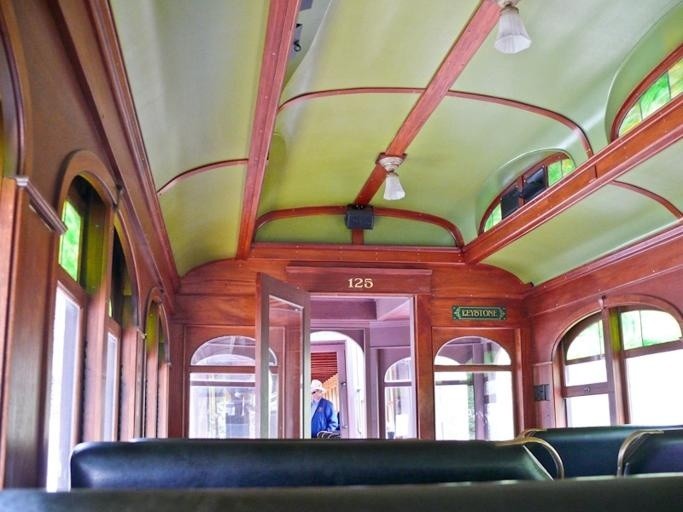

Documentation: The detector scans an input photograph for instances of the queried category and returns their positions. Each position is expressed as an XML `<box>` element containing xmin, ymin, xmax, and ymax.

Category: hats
<box><xmin>311</xmin><ymin>378</ymin><xmax>327</xmax><ymax>395</ymax></box>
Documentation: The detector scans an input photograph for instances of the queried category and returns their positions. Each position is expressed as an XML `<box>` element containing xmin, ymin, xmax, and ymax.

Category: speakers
<box><xmin>524</xmin><ymin>165</ymin><xmax>545</xmax><ymax>201</ymax></box>
<box><xmin>534</xmin><ymin>384</ymin><xmax>549</xmax><ymax>401</ymax></box>
<box><xmin>501</xmin><ymin>189</ymin><xmax>520</xmax><ymax>219</ymax></box>
<box><xmin>345</xmin><ymin>204</ymin><xmax>373</xmax><ymax>229</ymax></box>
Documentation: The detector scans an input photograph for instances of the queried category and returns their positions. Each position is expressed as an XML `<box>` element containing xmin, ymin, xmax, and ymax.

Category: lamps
<box><xmin>491</xmin><ymin>0</ymin><xmax>532</xmax><ymax>56</ymax></box>
<box><xmin>375</xmin><ymin>151</ymin><xmax>407</xmax><ymax>201</ymax></box>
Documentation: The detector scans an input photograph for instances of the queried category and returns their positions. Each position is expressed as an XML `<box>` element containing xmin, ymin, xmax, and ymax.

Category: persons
<box><xmin>308</xmin><ymin>378</ymin><xmax>339</xmax><ymax>440</ymax></box>
